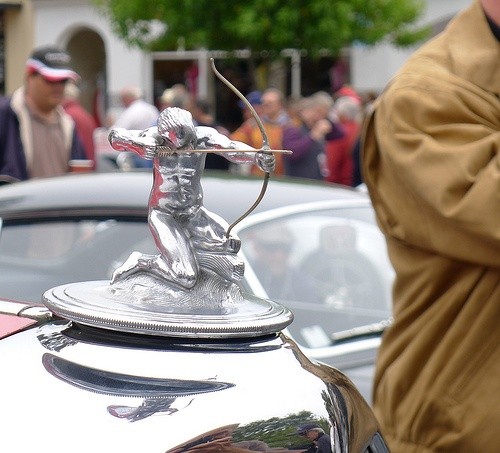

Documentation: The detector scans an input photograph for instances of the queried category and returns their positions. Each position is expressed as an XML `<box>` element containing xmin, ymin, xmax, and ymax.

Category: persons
<box><xmin>0</xmin><ymin>44</ymin><xmax>84</xmax><ymax>191</ymax></box>
<box><xmin>358</xmin><ymin>0</ymin><xmax>500</xmax><ymax>453</ymax></box>
<box><xmin>105</xmin><ymin>104</ymin><xmax>275</xmax><ymax>293</ymax></box>
<box><xmin>157</xmin><ymin>68</ymin><xmax>373</xmax><ymax>189</ymax></box>
<box><xmin>59</xmin><ymin>81</ymin><xmax>99</xmax><ymax>172</ymax></box>
<box><xmin>289</xmin><ymin>425</ymin><xmax>331</xmax><ymax>453</ymax></box>
<box><xmin>104</xmin><ymin>84</ymin><xmax>162</xmax><ymax>171</ymax></box>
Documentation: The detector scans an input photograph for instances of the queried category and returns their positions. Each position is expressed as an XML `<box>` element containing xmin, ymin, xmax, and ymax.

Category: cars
<box><xmin>0</xmin><ymin>167</ymin><xmax>398</xmax><ymax>453</ymax></box>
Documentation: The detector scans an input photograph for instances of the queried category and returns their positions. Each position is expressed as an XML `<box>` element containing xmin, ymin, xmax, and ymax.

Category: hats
<box><xmin>24</xmin><ymin>44</ymin><xmax>83</xmax><ymax>82</ymax></box>
<box><xmin>287</xmin><ymin>423</ymin><xmax>320</xmax><ymax>437</ymax></box>
<box><xmin>237</xmin><ymin>90</ymin><xmax>264</xmax><ymax>109</ymax></box>
